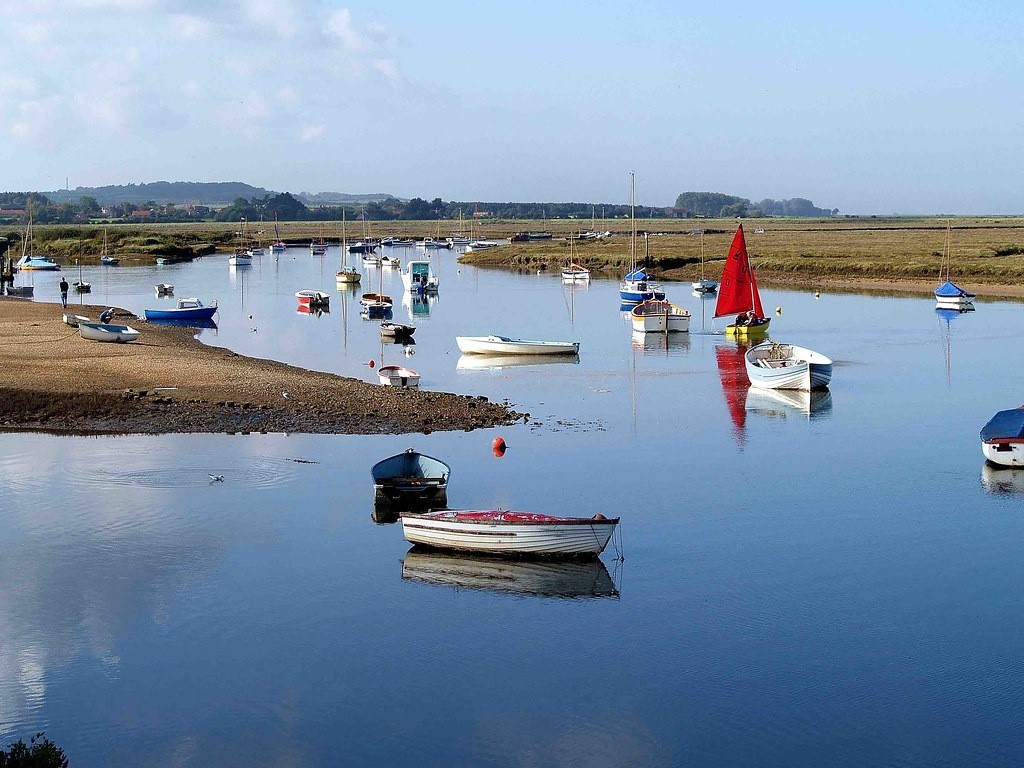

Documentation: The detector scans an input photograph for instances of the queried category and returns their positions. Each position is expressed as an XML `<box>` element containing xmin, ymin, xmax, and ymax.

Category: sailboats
<box><xmin>934</xmin><ymin>302</ymin><xmax>976</xmax><ymax>385</ymax></box>
<box><xmin>692</xmin><ymin>232</ymin><xmax>716</xmax><ymax>292</ymax></box>
<box><xmin>712</xmin><ymin>222</ymin><xmax>772</xmax><ymax>333</ymax></box>
<box><xmin>101</xmin><ymin>227</ymin><xmax>120</xmax><ymax>264</ymax></box>
<box><xmin>268</xmin><ymin>211</ymin><xmax>287</xmax><ymax>250</ymax></box>
<box><xmin>16</xmin><ymin>207</ymin><xmax>61</xmax><ymax>271</ymax></box>
<box><xmin>933</xmin><ymin>220</ymin><xmax>976</xmax><ymax>302</ymax></box>
<box><xmin>72</xmin><ymin>221</ymin><xmax>91</xmax><ymax>291</ymax></box>
<box><xmin>619</xmin><ymin>170</ymin><xmax>664</xmax><ymax>303</ymax></box>
<box><xmin>228</xmin><ymin>213</ymin><xmax>264</xmax><ymax>266</ymax></box>
<box><xmin>334</xmin><ymin>207</ymin><xmax>360</xmax><ymax>283</ymax></box>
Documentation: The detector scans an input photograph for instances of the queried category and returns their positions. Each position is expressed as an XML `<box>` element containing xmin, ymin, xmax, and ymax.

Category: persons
<box><xmin>735</xmin><ymin>311</ymin><xmax>760</xmax><ymax>327</ymax></box>
<box><xmin>99</xmin><ymin>308</ymin><xmax>115</xmax><ymax>324</ymax></box>
<box><xmin>59</xmin><ymin>277</ymin><xmax>68</xmax><ymax>308</ymax></box>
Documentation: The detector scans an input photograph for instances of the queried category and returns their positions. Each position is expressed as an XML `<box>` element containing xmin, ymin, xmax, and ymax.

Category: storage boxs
<box><xmin>63</xmin><ymin>313</ymin><xmax>91</xmax><ymax>325</ymax></box>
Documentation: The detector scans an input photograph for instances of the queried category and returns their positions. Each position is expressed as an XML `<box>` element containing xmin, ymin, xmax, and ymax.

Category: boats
<box><xmin>310</xmin><ymin>223</ymin><xmax>328</xmax><ymax>248</ymax></box>
<box><xmin>399</xmin><ymin>508</ymin><xmax>619</xmax><ymax>560</ymax></box>
<box><xmin>144</xmin><ymin>297</ymin><xmax>218</xmax><ymax>319</ymax></box>
<box><xmin>455</xmin><ymin>335</ymin><xmax>580</xmax><ymax>356</ymax></box>
<box><xmin>377</xmin><ymin>366</ymin><xmax>421</xmax><ymax>386</ymax></box>
<box><xmin>360</xmin><ymin>292</ymin><xmax>392</xmax><ymax>314</ymax></box>
<box><xmin>573</xmin><ymin>206</ymin><xmax>614</xmax><ymax>239</ymax></box>
<box><xmin>78</xmin><ymin>323</ymin><xmax>140</xmax><ymax>343</ymax></box>
<box><xmin>979</xmin><ymin>405</ymin><xmax>1024</xmax><ymax>466</ymax></box>
<box><xmin>370</xmin><ymin>447</ymin><xmax>451</xmax><ymax>504</ymax></box>
<box><xmin>364</xmin><ymin>253</ymin><xmax>439</xmax><ymax>291</ymax></box>
<box><xmin>155</xmin><ymin>283</ymin><xmax>174</xmax><ymax>293</ymax></box>
<box><xmin>6</xmin><ymin>287</ymin><xmax>33</xmax><ymax>295</ymax></box>
<box><xmin>744</xmin><ymin>340</ymin><xmax>832</xmax><ymax>392</ymax></box>
<box><xmin>561</xmin><ymin>231</ymin><xmax>591</xmax><ymax>279</ymax></box>
<box><xmin>294</xmin><ymin>290</ymin><xmax>330</xmax><ymax>306</ymax></box>
<box><xmin>378</xmin><ymin>323</ymin><xmax>416</xmax><ymax>337</ymax></box>
<box><xmin>311</xmin><ymin>248</ymin><xmax>325</xmax><ymax>255</ymax></box>
<box><xmin>629</xmin><ymin>298</ymin><xmax>691</xmax><ymax>333</ymax></box>
<box><xmin>350</xmin><ymin>209</ymin><xmax>551</xmax><ymax>253</ymax></box>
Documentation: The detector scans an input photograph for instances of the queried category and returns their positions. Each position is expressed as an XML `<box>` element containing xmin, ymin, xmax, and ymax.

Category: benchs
<box><xmin>376</xmin><ymin>477</ymin><xmax>445</xmax><ymax>483</ymax></box>
